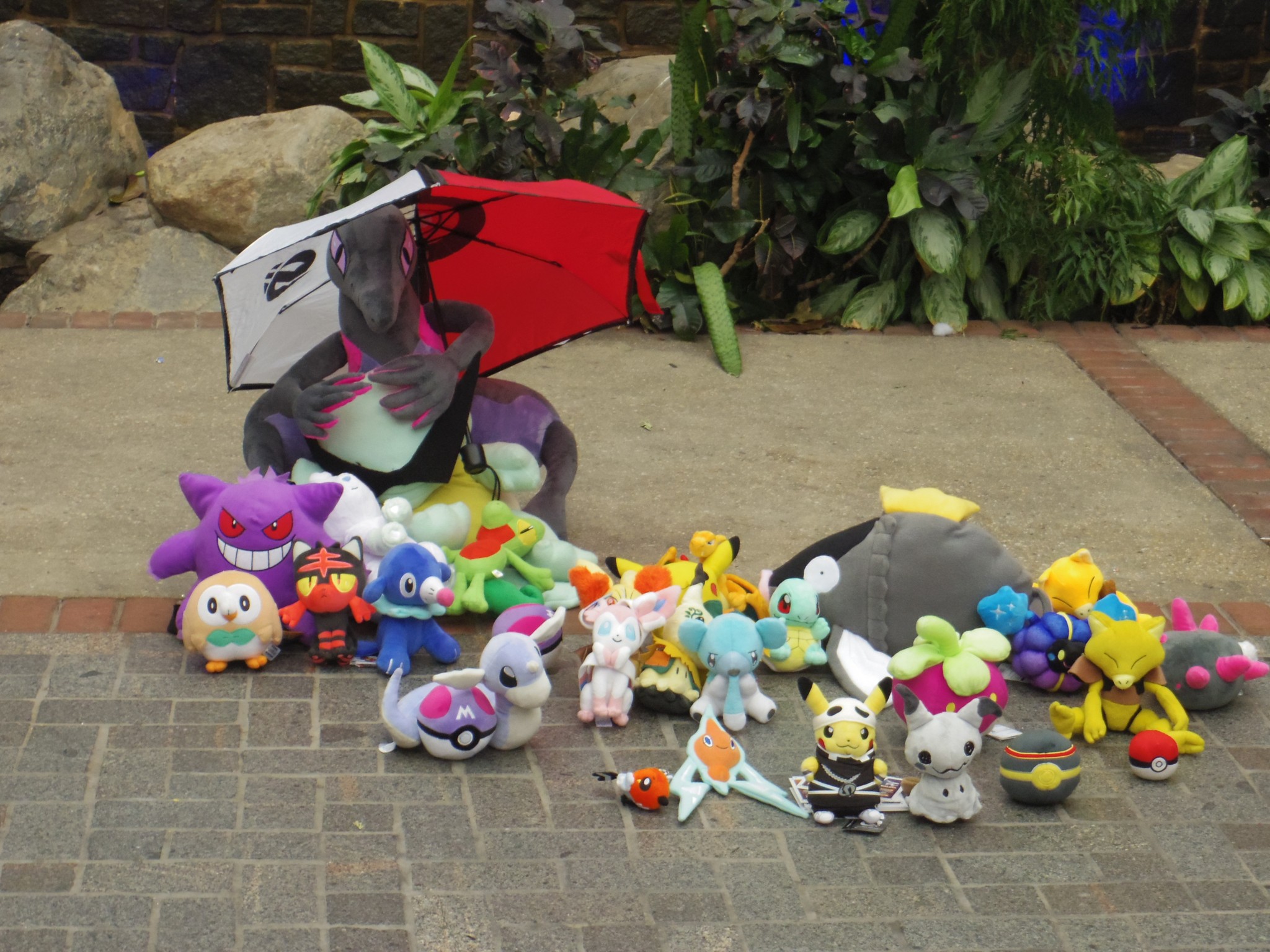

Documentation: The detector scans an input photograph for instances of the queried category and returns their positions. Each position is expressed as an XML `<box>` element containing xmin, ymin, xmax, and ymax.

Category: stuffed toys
<box><xmin>150</xmin><ymin>205</ymin><xmax>580</xmax><ymax>675</ymax></box>
<box><xmin>378</xmin><ymin>485</ymin><xmax>1270</xmax><ymax>837</ymax></box>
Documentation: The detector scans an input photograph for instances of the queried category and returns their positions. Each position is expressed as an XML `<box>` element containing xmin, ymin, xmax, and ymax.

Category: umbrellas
<box><xmin>212</xmin><ymin>165</ymin><xmax>663</xmax><ymax>395</ymax></box>
